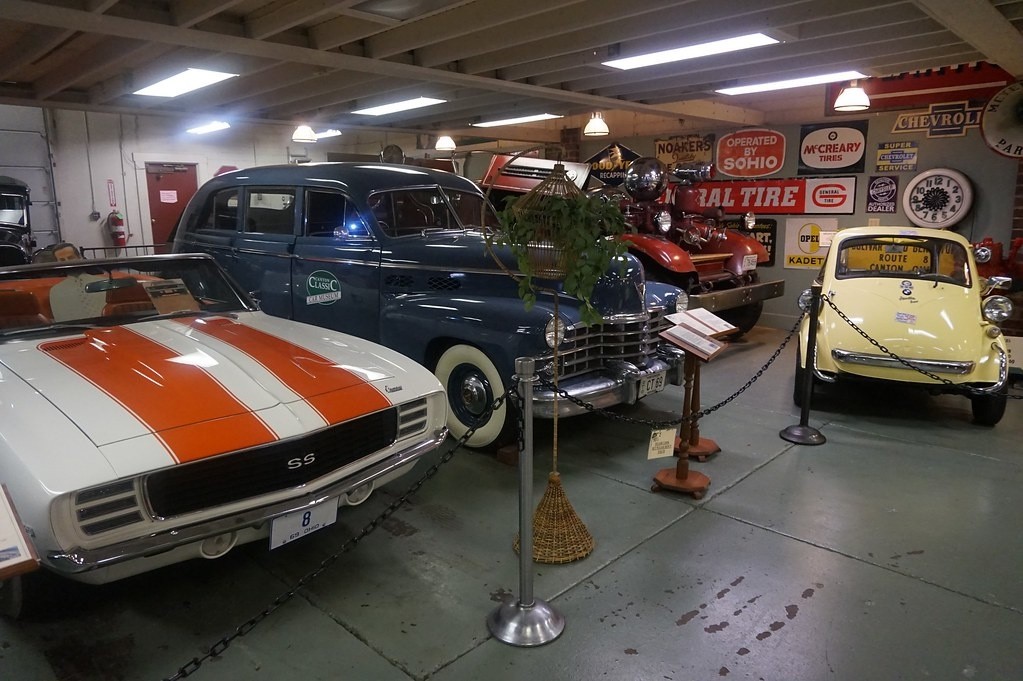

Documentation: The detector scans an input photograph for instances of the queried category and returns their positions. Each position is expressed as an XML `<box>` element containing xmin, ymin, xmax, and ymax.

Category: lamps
<box><xmin>583</xmin><ymin>111</ymin><xmax>609</xmax><ymax>137</ymax></box>
<box><xmin>291</xmin><ymin>126</ymin><xmax>317</xmax><ymax>143</ymax></box>
<box><xmin>833</xmin><ymin>80</ymin><xmax>871</xmax><ymax>112</ymax></box>
<box><xmin>434</xmin><ymin>136</ymin><xmax>457</xmax><ymax>150</ymax></box>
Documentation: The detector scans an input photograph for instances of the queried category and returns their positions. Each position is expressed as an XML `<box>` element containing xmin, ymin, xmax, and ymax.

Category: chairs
<box><xmin>103</xmin><ymin>284</ymin><xmax>155</xmax><ymax>315</ymax></box>
<box><xmin>0</xmin><ymin>290</ymin><xmax>47</xmax><ymax>326</ymax></box>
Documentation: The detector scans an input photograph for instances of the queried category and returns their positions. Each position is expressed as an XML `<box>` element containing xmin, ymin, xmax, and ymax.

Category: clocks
<box><xmin>902</xmin><ymin>168</ymin><xmax>972</xmax><ymax>228</ymax></box>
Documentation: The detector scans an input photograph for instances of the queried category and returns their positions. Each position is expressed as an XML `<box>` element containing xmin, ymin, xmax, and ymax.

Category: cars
<box><xmin>476</xmin><ymin>152</ymin><xmax>785</xmax><ymax>342</ymax></box>
<box><xmin>0</xmin><ymin>243</ymin><xmax>449</xmax><ymax>622</ymax></box>
<box><xmin>171</xmin><ymin>160</ymin><xmax>688</xmax><ymax>453</ymax></box>
<box><xmin>793</xmin><ymin>226</ymin><xmax>1013</xmax><ymax>426</ymax></box>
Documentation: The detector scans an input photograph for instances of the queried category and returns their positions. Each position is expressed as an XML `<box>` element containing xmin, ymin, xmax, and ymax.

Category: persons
<box><xmin>48</xmin><ymin>243</ymin><xmax>111</xmax><ymax>323</ymax></box>
